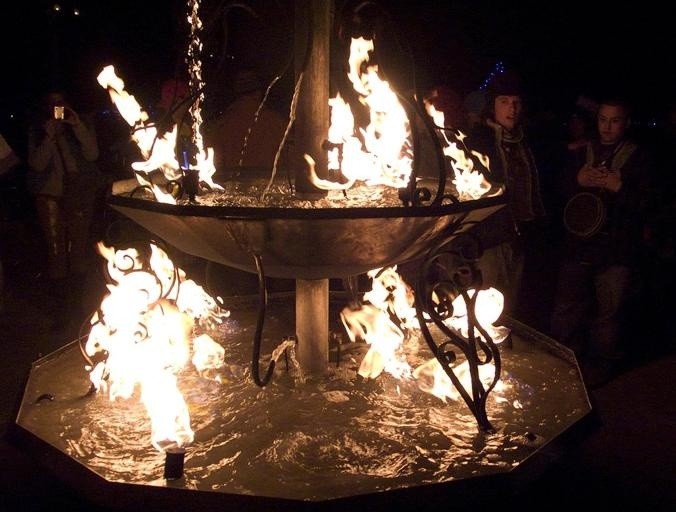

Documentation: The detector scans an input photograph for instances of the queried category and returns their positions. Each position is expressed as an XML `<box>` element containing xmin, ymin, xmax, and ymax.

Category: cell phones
<box><xmin>54</xmin><ymin>105</ymin><xmax>65</xmax><ymax>120</ymax></box>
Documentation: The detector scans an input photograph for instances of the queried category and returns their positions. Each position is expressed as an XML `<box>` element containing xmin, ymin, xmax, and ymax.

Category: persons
<box><xmin>29</xmin><ymin>88</ymin><xmax>100</xmax><ymax>290</ymax></box>
<box><xmin>201</xmin><ymin>69</ymin><xmax>290</xmax><ymax>171</ymax></box>
<box><xmin>153</xmin><ymin>80</ymin><xmax>210</xmax><ymax>156</ymax></box>
<box><xmin>540</xmin><ymin>100</ymin><xmax>649</xmax><ymax>368</ymax></box>
<box><xmin>548</xmin><ymin>92</ymin><xmax>608</xmax><ymax>202</ymax></box>
<box><xmin>470</xmin><ymin>85</ymin><xmax>550</xmax><ymax>318</ymax></box>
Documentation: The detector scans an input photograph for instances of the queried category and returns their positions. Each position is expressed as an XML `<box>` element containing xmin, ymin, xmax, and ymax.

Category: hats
<box><xmin>480</xmin><ymin>68</ymin><xmax>530</xmax><ymax>119</ymax></box>
